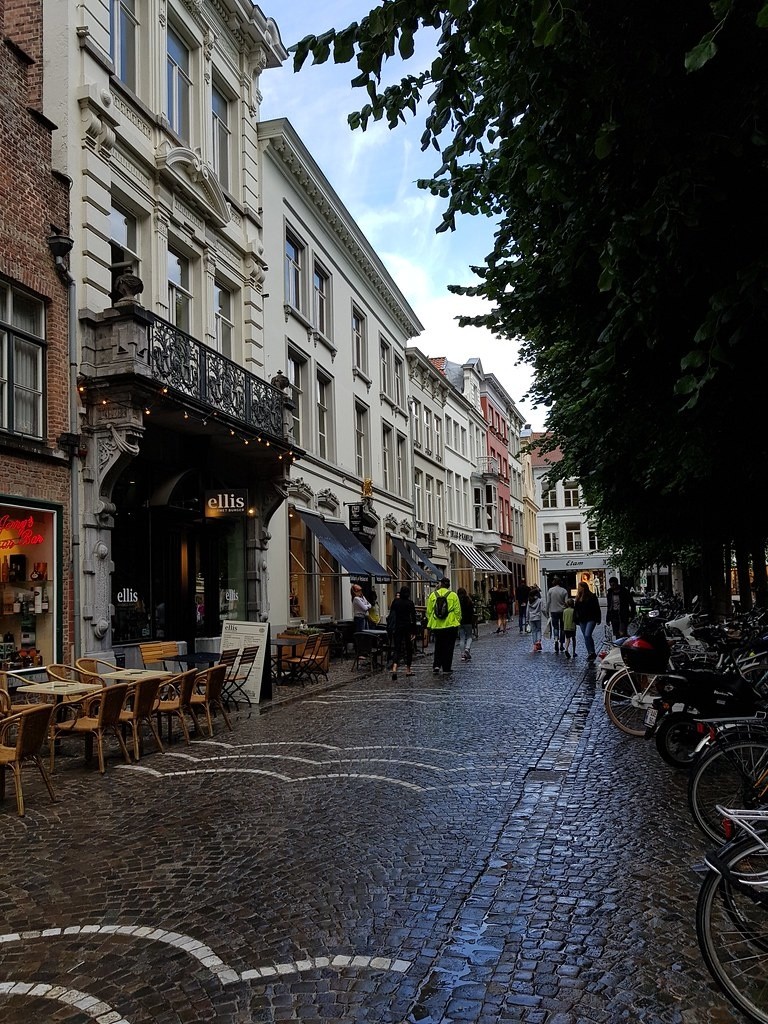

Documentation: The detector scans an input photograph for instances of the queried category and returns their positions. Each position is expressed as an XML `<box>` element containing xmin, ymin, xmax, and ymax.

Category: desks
<box><xmin>157</xmin><ymin>652</ymin><xmax>222</xmax><ymax>668</ymax></box>
<box><xmin>271</xmin><ymin>639</ymin><xmax>306</xmax><ymax>685</ymax></box>
<box><xmin>99</xmin><ymin>668</ymin><xmax>173</xmax><ymax>713</ymax></box>
<box><xmin>17</xmin><ymin>680</ymin><xmax>104</xmax><ymax>757</ymax></box>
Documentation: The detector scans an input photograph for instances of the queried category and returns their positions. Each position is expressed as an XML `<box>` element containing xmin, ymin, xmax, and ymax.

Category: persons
<box><xmin>489</xmin><ymin>583</ymin><xmax>515</xmax><ymax>634</ymax></box>
<box><xmin>427</xmin><ymin>577</ymin><xmax>462</xmax><ymax>674</ymax></box>
<box><xmin>386</xmin><ymin>586</ymin><xmax>416</xmax><ymax>680</ymax></box>
<box><xmin>582</xmin><ymin>573</ymin><xmax>593</xmax><ymax>592</ymax></box>
<box><xmin>606</xmin><ymin>577</ymin><xmax>636</xmax><ymax>640</ymax></box>
<box><xmin>526</xmin><ymin>579</ymin><xmax>568</xmax><ymax>651</ymax></box>
<box><xmin>515</xmin><ymin>578</ymin><xmax>542</xmax><ymax>634</ymax></box>
<box><xmin>195</xmin><ymin>599</ymin><xmax>205</xmax><ymax>623</ymax></box>
<box><xmin>350</xmin><ymin>585</ymin><xmax>381</xmax><ymax>660</ymax></box>
<box><xmin>594</xmin><ymin>574</ymin><xmax>601</xmax><ymax>597</ymax></box>
<box><xmin>563</xmin><ymin>600</ymin><xmax>578</xmax><ymax>658</ymax></box>
<box><xmin>457</xmin><ymin>587</ymin><xmax>474</xmax><ymax>662</ymax></box>
<box><xmin>572</xmin><ymin>582</ymin><xmax>601</xmax><ymax>659</ymax></box>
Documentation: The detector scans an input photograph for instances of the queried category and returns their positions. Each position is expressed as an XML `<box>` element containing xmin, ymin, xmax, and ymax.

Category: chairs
<box><xmin>49</xmin><ymin>682</ymin><xmax>132</xmax><ymax>775</ymax></box>
<box><xmin>76</xmin><ymin>658</ymin><xmax>125</xmax><ymax>685</ymax></box>
<box><xmin>153</xmin><ymin>668</ymin><xmax>205</xmax><ymax>746</ymax></box>
<box><xmin>1</xmin><ymin>671</ymin><xmax>49</xmax><ymax>749</ymax></box>
<box><xmin>298</xmin><ymin>631</ymin><xmax>334</xmax><ymax>684</ymax></box>
<box><xmin>193</xmin><ymin>649</ymin><xmax>240</xmax><ymax>718</ymax></box>
<box><xmin>222</xmin><ymin>645</ymin><xmax>260</xmax><ymax>712</ymax></box>
<box><xmin>188</xmin><ymin>664</ymin><xmax>233</xmax><ymax>739</ymax></box>
<box><xmin>351</xmin><ymin>632</ymin><xmax>385</xmax><ymax>674</ymax></box>
<box><xmin>281</xmin><ymin>634</ymin><xmax>319</xmax><ymax>688</ymax></box>
<box><xmin>138</xmin><ymin>641</ymin><xmax>184</xmax><ymax>676</ymax></box>
<box><xmin>115</xmin><ymin>676</ymin><xmax>167</xmax><ymax>760</ymax></box>
<box><xmin>1</xmin><ymin>704</ymin><xmax>58</xmax><ymax>818</ymax></box>
<box><xmin>47</xmin><ymin>664</ymin><xmax>107</xmax><ymax>722</ymax></box>
<box><xmin>270</xmin><ymin>651</ymin><xmax>289</xmax><ymax>680</ymax></box>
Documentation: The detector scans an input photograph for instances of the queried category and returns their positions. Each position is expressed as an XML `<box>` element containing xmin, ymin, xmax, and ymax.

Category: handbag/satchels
<box><xmin>526</xmin><ymin>624</ymin><xmax>531</xmax><ymax>632</ymax></box>
<box><xmin>543</xmin><ymin>617</ymin><xmax>552</xmax><ymax>641</ymax></box>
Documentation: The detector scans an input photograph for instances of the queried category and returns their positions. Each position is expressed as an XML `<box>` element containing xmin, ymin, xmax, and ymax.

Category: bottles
<box><xmin>41</xmin><ymin>589</ymin><xmax>49</xmax><ymax>612</ymax></box>
<box><xmin>1</xmin><ymin>555</ymin><xmax>9</xmax><ymax>583</ymax></box>
<box><xmin>12</xmin><ymin>587</ymin><xmax>34</xmax><ymax>613</ymax></box>
<box><xmin>30</xmin><ymin>562</ymin><xmax>42</xmax><ymax>581</ymax></box>
<box><xmin>22</xmin><ymin>650</ymin><xmax>43</xmax><ymax>668</ymax></box>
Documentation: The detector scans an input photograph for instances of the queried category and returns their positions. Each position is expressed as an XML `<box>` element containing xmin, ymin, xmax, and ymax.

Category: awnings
<box><xmin>289</xmin><ymin>510</ymin><xmax>391</xmax><ymax>577</ymax></box>
<box><xmin>452</xmin><ymin>543</ymin><xmax>511</xmax><ymax>574</ymax></box>
<box><xmin>387</xmin><ymin>537</ymin><xmax>446</xmax><ymax>581</ymax></box>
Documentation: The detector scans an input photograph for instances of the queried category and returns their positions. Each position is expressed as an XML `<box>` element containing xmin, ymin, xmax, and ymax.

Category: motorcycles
<box><xmin>621</xmin><ymin>633</ymin><xmax>768</xmax><ymax>768</ymax></box>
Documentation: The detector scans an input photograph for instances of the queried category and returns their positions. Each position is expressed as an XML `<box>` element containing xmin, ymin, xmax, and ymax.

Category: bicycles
<box><xmin>598</xmin><ymin>588</ymin><xmax>768</xmax><ymax>1023</ymax></box>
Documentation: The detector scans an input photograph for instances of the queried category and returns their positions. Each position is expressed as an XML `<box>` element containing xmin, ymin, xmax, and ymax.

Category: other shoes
<box><xmin>464</xmin><ymin>648</ymin><xmax>471</xmax><ymax>659</ymax></box>
<box><xmin>392</xmin><ymin>673</ymin><xmax>397</xmax><ymax>680</ymax></box>
<box><xmin>406</xmin><ymin>672</ymin><xmax>416</xmax><ymax>676</ymax></box>
<box><xmin>534</xmin><ymin>640</ymin><xmax>542</xmax><ymax>651</ymax></box>
<box><xmin>461</xmin><ymin>655</ymin><xmax>466</xmax><ymax>660</ymax></box>
<box><xmin>573</xmin><ymin>653</ymin><xmax>578</xmax><ymax>657</ymax></box>
<box><xmin>433</xmin><ymin>667</ymin><xmax>440</xmax><ymax>674</ymax></box>
<box><xmin>564</xmin><ymin>651</ymin><xmax>571</xmax><ymax>658</ymax></box>
<box><xmin>359</xmin><ymin>656</ymin><xmax>366</xmax><ymax>659</ymax></box>
<box><xmin>519</xmin><ymin>631</ymin><xmax>523</xmax><ymax>634</ymax></box>
<box><xmin>586</xmin><ymin>653</ymin><xmax>597</xmax><ymax>662</ymax></box>
<box><xmin>560</xmin><ymin>646</ymin><xmax>565</xmax><ymax>651</ymax></box>
<box><xmin>503</xmin><ymin>630</ymin><xmax>508</xmax><ymax>634</ymax></box>
<box><xmin>555</xmin><ymin>641</ymin><xmax>559</xmax><ymax>652</ymax></box>
<box><xmin>443</xmin><ymin>669</ymin><xmax>453</xmax><ymax>674</ymax></box>
<box><xmin>496</xmin><ymin>628</ymin><xmax>500</xmax><ymax>633</ymax></box>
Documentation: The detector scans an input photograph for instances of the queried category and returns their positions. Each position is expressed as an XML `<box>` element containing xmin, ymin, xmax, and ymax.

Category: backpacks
<box><xmin>434</xmin><ymin>591</ymin><xmax>454</xmax><ymax>620</ymax></box>
